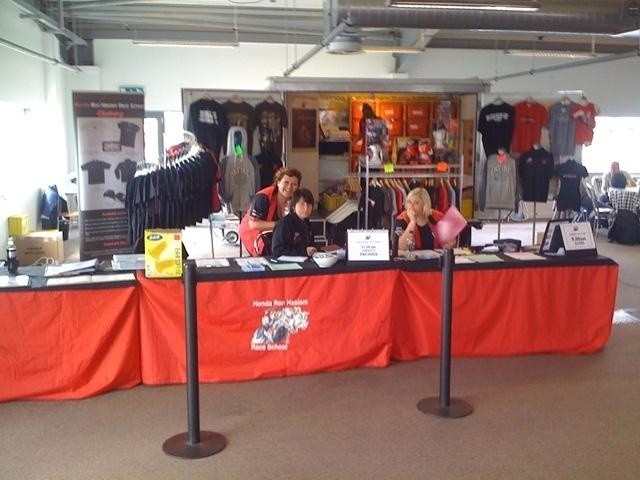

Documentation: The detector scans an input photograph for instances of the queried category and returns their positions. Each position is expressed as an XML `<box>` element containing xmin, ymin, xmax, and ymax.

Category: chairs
<box><xmin>584</xmin><ymin>176</ymin><xmax>640</xmax><ymax>242</ymax></box>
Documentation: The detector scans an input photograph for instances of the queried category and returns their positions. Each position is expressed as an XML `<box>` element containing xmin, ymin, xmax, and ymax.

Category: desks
<box><xmin>0</xmin><ymin>244</ymin><xmax>619</xmax><ymax>399</ymax></box>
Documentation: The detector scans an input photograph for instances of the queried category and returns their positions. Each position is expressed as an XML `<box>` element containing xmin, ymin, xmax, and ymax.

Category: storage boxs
<box><xmin>14</xmin><ymin>231</ymin><xmax>65</xmax><ymax>266</ymax></box>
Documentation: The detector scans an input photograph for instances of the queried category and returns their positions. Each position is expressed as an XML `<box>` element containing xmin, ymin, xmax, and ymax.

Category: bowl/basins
<box><xmin>311</xmin><ymin>250</ymin><xmax>346</xmax><ymax>268</ymax></box>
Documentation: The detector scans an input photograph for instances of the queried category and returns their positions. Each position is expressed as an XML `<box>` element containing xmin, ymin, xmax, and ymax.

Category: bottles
<box><xmin>7</xmin><ymin>237</ymin><xmax>17</xmax><ymax>272</ymax></box>
<box><xmin>404</xmin><ymin>230</ymin><xmax>416</xmax><ymax>261</ymax></box>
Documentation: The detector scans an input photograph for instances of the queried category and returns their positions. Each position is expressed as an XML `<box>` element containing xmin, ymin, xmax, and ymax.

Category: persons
<box><xmin>398</xmin><ymin>136</ymin><xmax>436</xmax><ymax>179</ymax></box>
<box><xmin>271</xmin><ymin>187</ymin><xmax>318</xmax><ymax>256</ymax></box>
<box><xmin>238</xmin><ymin>166</ymin><xmax>303</xmax><ymax>256</ymax></box>
<box><xmin>391</xmin><ymin>187</ymin><xmax>458</xmax><ymax>253</ymax></box>
<box><xmin>598</xmin><ymin>161</ymin><xmax>637</xmax><ymax>228</ymax></box>
<box><xmin>352</xmin><ymin>101</ymin><xmax>389</xmax><ymax>192</ymax></box>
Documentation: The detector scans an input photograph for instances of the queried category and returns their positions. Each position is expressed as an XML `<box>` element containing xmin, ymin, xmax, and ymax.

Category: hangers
<box><xmin>481</xmin><ymin>91</ymin><xmax>596</xmax><ymax>109</ymax></box>
<box><xmin>362</xmin><ymin>165</ymin><xmax>461</xmax><ymax>198</ymax></box>
<box><xmin>191</xmin><ymin>89</ymin><xmax>285</xmax><ymax>112</ymax></box>
